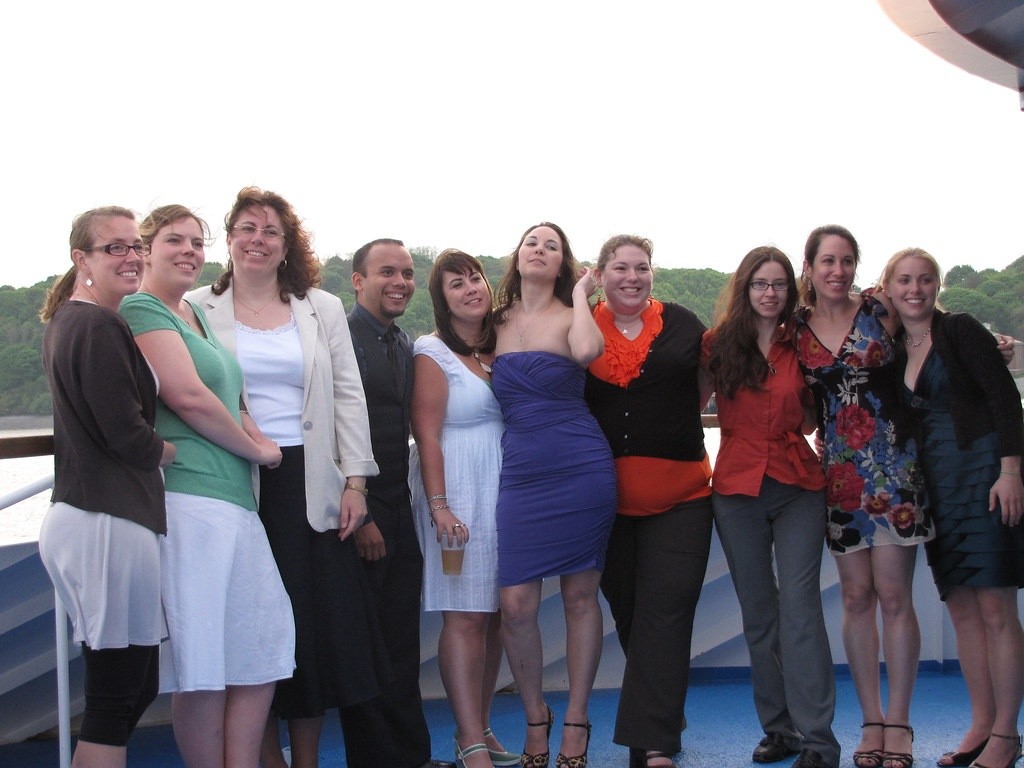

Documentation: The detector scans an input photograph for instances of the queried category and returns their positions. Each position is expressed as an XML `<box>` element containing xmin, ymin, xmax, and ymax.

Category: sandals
<box><xmin>853</xmin><ymin>722</ymin><xmax>884</xmax><ymax>768</ymax></box>
<box><xmin>884</xmin><ymin>724</ymin><xmax>914</xmax><ymax>768</ymax></box>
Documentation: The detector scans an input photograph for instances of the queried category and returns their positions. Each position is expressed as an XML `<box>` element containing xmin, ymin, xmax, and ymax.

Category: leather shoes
<box><xmin>423</xmin><ymin>758</ymin><xmax>456</xmax><ymax>768</ymax></box>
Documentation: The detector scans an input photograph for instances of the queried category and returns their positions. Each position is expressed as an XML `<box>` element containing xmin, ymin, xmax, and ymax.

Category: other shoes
<box><xmin>752</xmin><ymin>738</ymin><xmax>800</xmax><ymax>763</ymax></box>
<box><xmin>455</xmin><ymin>743</ymin><xmax>494</xmax><ymax>768</ymax></box>
<box><xmin>453</xmin><ymin>726</ymin><xmax>521</xmax><ymax>766</ymax></box>
<box><xmin>791</xmin><ymin>759</ymin><xmax>804</xmax><ymax>768</ymax></box>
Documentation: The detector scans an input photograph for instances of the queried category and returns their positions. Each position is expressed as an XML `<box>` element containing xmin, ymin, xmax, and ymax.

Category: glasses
<box><xmin>749</xmin><ymin>281</ymin><xmax>791</xmax><ymax>291</ymax></box>
<box><xmin>82</xmin><ymin>243</ymin><xmax>151</xmax><ymax>256</ymax></box>
<box><xmin>233</xmin><ymin>224</ymin><xmax>286</xmax><ymax>240</ymax></box>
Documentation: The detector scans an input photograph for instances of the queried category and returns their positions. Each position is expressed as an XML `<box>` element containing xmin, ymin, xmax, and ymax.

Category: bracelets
<box><xmin>999</xmin><ymin>470</ymin><xmax>1021</xmax><ymax>476</ymax></box>
<box><xmin>239</xmin><ymin>410</ymin><xmax>250</xmax><ymax>418</ymax></box>
<box><xmin>427</xmin><ymin>495</ymin><xmax>447</xmax><ymax>503</ymax></box>
<box><xmin>345</xmin><ymin>484</ymin><xmax>369</xmax><ymax>496</ymax></box>
<box><xmin>428</xmin><ymin>505</ymin><xmax>450</xmax><ymax>517</ymax></box>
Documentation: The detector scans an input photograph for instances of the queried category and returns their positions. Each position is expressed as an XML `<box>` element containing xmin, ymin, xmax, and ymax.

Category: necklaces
<box><xmin>517</xmin><ymin>301</ymin><xmax>553</xmax><ymax>341</ymax></box>
<box><xmin>613</xmin><ymin>319</ymin><xmax>644</xmax><ymax>333</ymax></box>
<box><xmin>905</xmin><ymin>327</ymin><xmax>930</xmax><ymax>347</ymax></box>
<box><xmin>79</xmin><ymin>281</ymin><xmax>104</xmax><ymax>308</ymax></box>
<box><xmin>472</xmin><ymin>350</ymin><xmax>492</xmax><ymax>374</ymax></box>
<box><xmin>233</xmin><ymin>293</ymin><xmax>282</xmax><ymax>315</ymax></box>
<box><xmin>182</xmin><ymin>308</ymin><xmax>192</xmax><ymax>327</ymax></box>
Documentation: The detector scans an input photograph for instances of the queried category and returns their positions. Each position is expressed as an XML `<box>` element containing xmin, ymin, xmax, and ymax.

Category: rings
<box><xmin>453</xmin><ymin>524</ymin><xmax>460</xmax><ymax>529</ymax></box>
<box><xmin>461</xmin><ymin>524</ymin><xmax>465</xmax><ymax>529</ymax></box>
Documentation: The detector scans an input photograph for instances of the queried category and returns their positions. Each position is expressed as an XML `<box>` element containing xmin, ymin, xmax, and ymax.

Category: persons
<box><xmin>694</xmin><ymin>247</ymin><xmax>843</xmax><ymax>768</ymax></box>
<box><xmin>38</xmin><ymin>204</ymin><xmax>175</xmax><ymax>768</ymax></box>
<box><xmin>180</xmin><ymin>186</ymin><xmax>380</xmax><ymax>768</ymax></box>
<box><xmin>785</xmin><ymin>226</ymin><xmax>1024</xmax><ymax>768</ymax></box>
<box><xmin>412</xmin><ymin>222</ymin><xmax>712</xmax><ymax>768</ymax></box>
<box><xmin>314</xmin><ymin>238</ymin><xmax>433</xmax><ymax>768</ymax></box>
<box><xmin>117</xmin><ymin>205</ymin><xmax>282</xmax><ymax>768</ymax></box>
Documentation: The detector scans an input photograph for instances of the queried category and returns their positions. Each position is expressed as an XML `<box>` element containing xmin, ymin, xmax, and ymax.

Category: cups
<box><xmin>440</xmin><ymin>530</ymin><xmax>465</xmax><ymax>576</ymax></box>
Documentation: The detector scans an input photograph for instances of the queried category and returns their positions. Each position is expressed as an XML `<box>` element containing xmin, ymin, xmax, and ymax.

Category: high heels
<box><xmin>556</xmin><ymin>719</ymin><xmax>592</xmax><ymax>768</ymax></box>
<box><xmin>629</xmin><ymin>746</ymin><xmax>675</xmax><ymax>768</ymax></box>
<box><xmin>519</xmin><ymin>704</ymin><xmax>554</xmax><ymax>768</ymax></box>
<box><xmin>937</xmin><ymin>736</ymin><xmax>990</xmax><ymax>768</ymax></box>
<box><xmin>967</xmin><ymin>733</ymin><xmax>1022</xmax><ymax>768</ymax></box>
